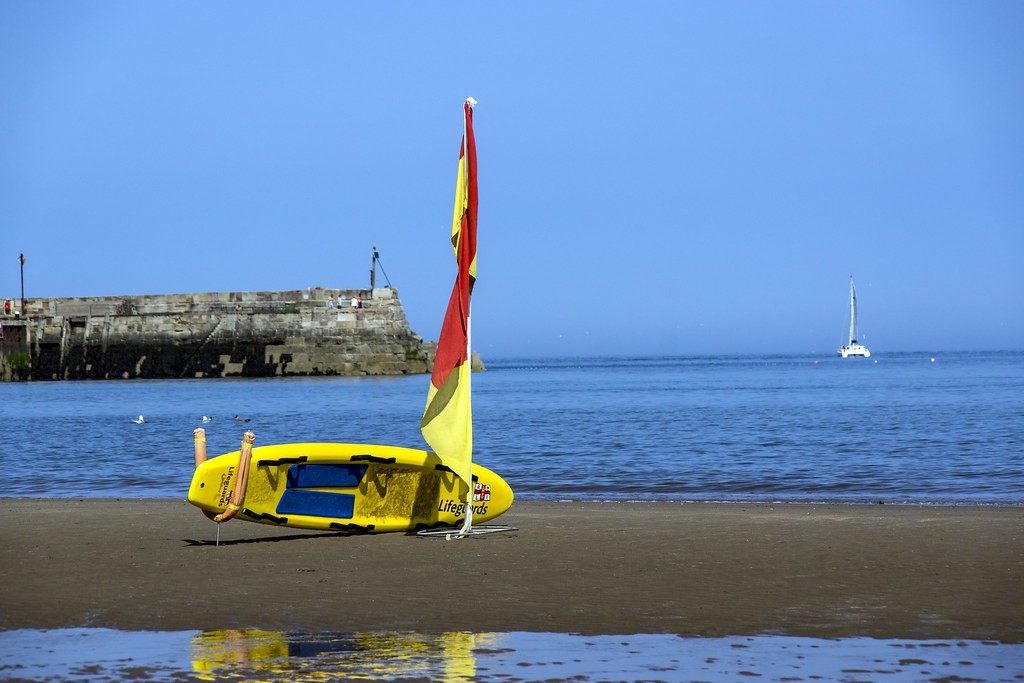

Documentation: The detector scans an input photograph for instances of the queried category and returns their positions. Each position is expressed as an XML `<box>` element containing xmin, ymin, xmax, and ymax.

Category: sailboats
<box><xmin>836</xmin><ymin>274</ymin><xmax>871</xmax><ymax>359</ymax></box>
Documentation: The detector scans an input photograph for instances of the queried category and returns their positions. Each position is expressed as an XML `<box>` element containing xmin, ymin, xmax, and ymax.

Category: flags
<box><xmin>419</xmin><ymin>102</ymin><xmax>479</xmax><ymax>504</ymax></box>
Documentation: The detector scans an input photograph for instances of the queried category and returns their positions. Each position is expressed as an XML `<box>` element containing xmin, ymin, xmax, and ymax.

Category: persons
<box><xmin>337</xmin><ymin>294</ymin><xmax>342</xmax><ymax>309</ymax></box>
<box><xmin>328</xmin><ymin>294</ymin><xmax>335</xmax><ymax>310</ymax></box>
<box><xmin>5</xmin><ymin>299</ymin><xmax>11</xmax><ymax>316</ymax></box>
<box><xmin>351</xmin><ymin>293</ymin><xmax>363</xmax><ymax>309</ymax></box>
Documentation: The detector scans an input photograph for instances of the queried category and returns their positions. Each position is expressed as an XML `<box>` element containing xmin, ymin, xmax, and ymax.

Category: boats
<box><xmin>187</xmin><ymin>427</ymin><xmax>515</xmax><ymax>535</ymax></box>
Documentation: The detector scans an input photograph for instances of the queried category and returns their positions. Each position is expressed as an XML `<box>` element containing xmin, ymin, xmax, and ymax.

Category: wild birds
<box><xmin>234</xmin><ymin>414</ymin><xmax>253</xmax><ymax>423</ymax></box>
<box><xmin>201</xmin><ymin>416</ymin><xmax>214</xmax><ymax>422</ymax></box>
<box><xmin>133</xmin><ymin>415</ymin><xmax>145</xmax><ymax>423</ymax></box>
